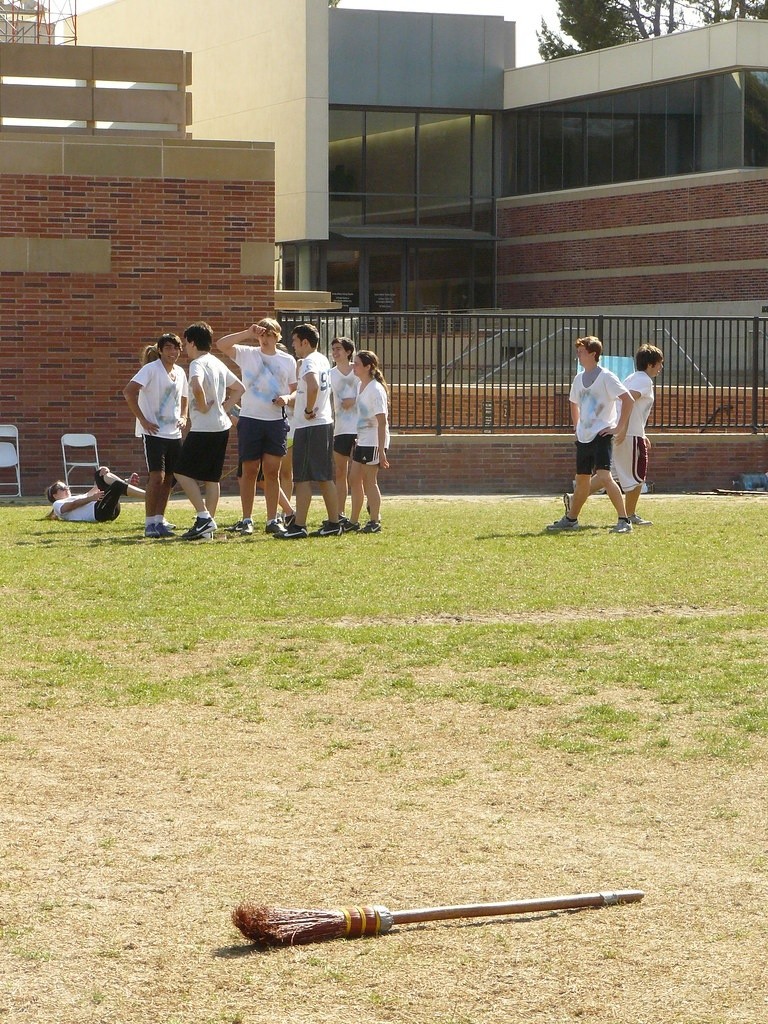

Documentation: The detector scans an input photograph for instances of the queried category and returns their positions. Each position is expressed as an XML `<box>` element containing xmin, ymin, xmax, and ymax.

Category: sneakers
<box><xmin>145</xmin><ymin>522</ymin><xmax>176</xmax><ymax>537</ymax></box>
<box><xmin>323</xmin><ymin>514</ymin><xmax>347</xmax><ymax>525</ymax></box>
<box><xmin>179</xmin><ymin>516</ymin><xmax>218</xmax><ymax>540</ymax></box>
<box><xmin>563</xmin><ymin>493</ymin><xmax>574</xmax><ymax>517</ymax></box>
<box><xmin>266</xmin><ymin>522</ymin><xmax>288</xmax><ymax>533</ymax></box>
<box><xmin>308</xmin><ymin>523</ymin><xmax>342</xmax><ymax>538</ymax></box>
<box><xmin>609</xmin><ymin>519</ymin><xmax>633</xmax><ymax>534</ymax></box>
<box><xmin>163</xmin><ymin>518</ymin><xmax>176</xmax><ymax>529</ymax></box>
<box><xmin>356</xmin><ymin>521</ymin><xmax>381</xmax><ymax>534</ymax></box>
<box><xmin>126</xmin><ymin>473</ymin><xmax>139</xmax><ymax>487</ymax></box>
<box><xmin>241</xmin><ymin>522</ymin><xmax>253</xmax><ymax>535</ymax></box>
<box><xmin>628</xmin><ymin>514</ymin><xmax>653</xmax><ymax>526</ymax></box>
<box><xmin>546</xmin><ymin>515</ymin><xmax>579</xmax><ymax>531</ymax></box>
<box><xmin>283</xmin><ymin>515</ymin><xmax>296</xmax><ymax>529</ymax></box>
<box><xmin>274</xmin><ymin>524</ymin><xmax>308</xmax><ymax>539</ymax></box>
<box><xmin>223</xmin><ymin>521</ymin><xmax>244</xmax><ymax>531</ymax></box>
<box><xmin>341</xmin><ymin>521</ymin><xmax>360</xmax><ymax>533</ymax></box>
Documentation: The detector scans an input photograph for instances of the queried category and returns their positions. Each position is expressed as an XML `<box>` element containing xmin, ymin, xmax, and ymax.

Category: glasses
<box><xmin>56</xmin><ymin>486</ymin><xmax>69</xmax><ymax>490</ymax></box>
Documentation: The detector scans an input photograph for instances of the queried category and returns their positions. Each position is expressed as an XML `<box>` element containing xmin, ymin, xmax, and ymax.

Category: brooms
<box><xmin>230</xmin><ymin>887</ymin><xmax>645</xmax><ymax>953</ymax></box>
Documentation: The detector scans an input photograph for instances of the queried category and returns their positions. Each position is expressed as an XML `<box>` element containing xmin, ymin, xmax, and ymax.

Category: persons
<box><xmin>547</xmin><ymin>336</ymin><xmax>633</xmax><ymax>534</ymax></box>
<box><xmin>47</xmin><ymin>466</ymin><xmax>146</xmax><ymax>521</ymax></box>
<box><xmin>563</xmin><ymin>344</ymin><xmax>664</xmax><ymax>526</ymax></box>
<box><xmin>123</xmin><ymin>318</ymin><xmax>391</xmax><ymax>540</ymax></box>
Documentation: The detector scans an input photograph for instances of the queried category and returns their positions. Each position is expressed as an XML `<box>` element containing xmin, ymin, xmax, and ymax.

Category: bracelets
<box><xmin>304</xmin><ymin>408</ymin><xmax>313</xmax><ymax>414</ymax></box>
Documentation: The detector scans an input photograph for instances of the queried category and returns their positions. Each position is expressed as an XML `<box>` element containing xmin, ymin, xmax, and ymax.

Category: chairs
<box><xmin>61</xmin><ymin>434</ymin><xmax>101</xmax><ymax>488</ymax></box>
<box><xmin>0</xmin><ymin>425</ymin><xmax>22</xmax><ymax>498</ymax></box>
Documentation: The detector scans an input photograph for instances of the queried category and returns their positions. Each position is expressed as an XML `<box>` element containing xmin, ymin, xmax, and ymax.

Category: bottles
<box><xmin>731</xmin><ymin>472</ymin><xmax>768</xmax><ymax>491</ymax></box>
